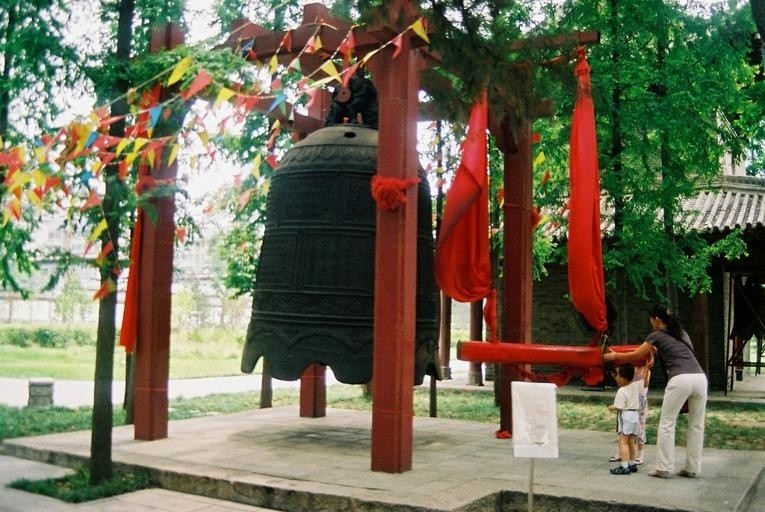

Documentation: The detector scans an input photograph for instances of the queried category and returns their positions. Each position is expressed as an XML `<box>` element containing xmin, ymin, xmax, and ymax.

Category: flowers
<box><xmin>531</xmin><ymin>206</ymin><xmax>540</xmax><ymax>230</ymax></box>
<box><xmin>369</xmin><ymin>172</ymin><xmax>407</xmax><ymax>211</ymax></box>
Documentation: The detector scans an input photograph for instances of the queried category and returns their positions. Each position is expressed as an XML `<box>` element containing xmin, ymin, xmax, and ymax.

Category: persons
<box><xmin>608</xmin><ymin>345</ymin><xmax>658</xmax><ymax>465</ymax></box>
<box><xmin>600</xmin><ymin>305</ymin><xmax>710</xmax><ymax>480</ymax></box>
<box><xmin>606</xmin><ymin>360</ymin><xmax>652</xmax><ymax>475</ymax></box>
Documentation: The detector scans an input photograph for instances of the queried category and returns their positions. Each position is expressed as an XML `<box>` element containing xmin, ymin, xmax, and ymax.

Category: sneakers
<box><xmin>674</xmin><ymin>467</ymin><xmax>698</xmax><ymax>479</ymax></box>
<box><xmin>648</xmin><ymin>469</ymin><xmax>670</xmax><ymax>481</ymax></box>
<box><xmin>608</xmin><ymin>450</ymin><xmax>644</xmax><ymax>478</ymax></box>
